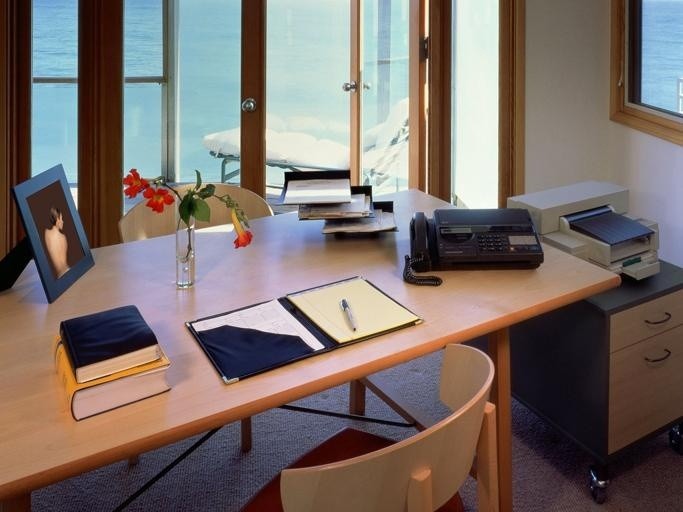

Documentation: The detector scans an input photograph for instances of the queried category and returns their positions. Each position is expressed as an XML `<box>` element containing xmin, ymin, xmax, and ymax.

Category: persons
<box><xmin>44</xmin><ymin>206</ymin><xmax>71</xmax><ymax>280</ymax></box>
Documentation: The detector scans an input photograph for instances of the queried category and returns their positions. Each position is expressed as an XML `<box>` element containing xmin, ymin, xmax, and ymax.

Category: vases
<box><xmin>174</xmin><ymin>194</ymin><xmax>195</xmax><ymax>288</ymax></box>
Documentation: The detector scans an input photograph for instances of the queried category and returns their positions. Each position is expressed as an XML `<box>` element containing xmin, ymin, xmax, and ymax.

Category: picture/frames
<box><xmin>0</xmin><ymin>163</ymin><xmax>95</xmax><ymax>304</ymax></box>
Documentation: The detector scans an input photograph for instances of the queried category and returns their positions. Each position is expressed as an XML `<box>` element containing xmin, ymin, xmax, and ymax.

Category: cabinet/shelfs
<box><xmin>463</xmin><ymin>259</ymin><xmax>683</xmax><ymax>504</ymax></box>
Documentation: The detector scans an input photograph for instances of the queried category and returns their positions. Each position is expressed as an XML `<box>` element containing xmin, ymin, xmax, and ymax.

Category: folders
<box><xmin>184</xmin><ymin>276</ymin><xmax>424</xmax><ymax>385</ymax></box>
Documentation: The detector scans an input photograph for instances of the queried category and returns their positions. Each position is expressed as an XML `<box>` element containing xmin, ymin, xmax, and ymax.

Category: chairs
<box><xmin>238</xmin><ymin>343</ymin><xmax>500</xmax><ymax>511</ymax></box>
<box><xmin>117</xmin><ymin>182</ymin><xmax>274</xmax><ymax>244</ymax></box>
<box><xmin>205</xmin><ymin>96</ymin><xmax>409</xmax><ymax>186</ymax></box>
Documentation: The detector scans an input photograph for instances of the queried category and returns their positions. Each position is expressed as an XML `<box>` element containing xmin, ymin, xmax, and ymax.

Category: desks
<box><xmin>1</xmin><ymin>188</ymin><xmax>621</xmax><ymax>512</ymax></box>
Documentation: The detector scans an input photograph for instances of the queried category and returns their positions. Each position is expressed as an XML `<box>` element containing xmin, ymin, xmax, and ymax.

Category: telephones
<box><xmin>410</xmin><ymin>209</ymin><xmax>543</xmax><ymax>272</ymax></box>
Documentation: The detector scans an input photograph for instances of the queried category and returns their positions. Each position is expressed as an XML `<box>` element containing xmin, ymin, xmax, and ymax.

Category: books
<box><xmin>53</xmin><ymin>335</ymin><xmax>171</xmax><ymax>422</ymax></box>
<box><xmin>60</xmin><ymin>306</ymin><xmax>162</xmax><ymax>383</ymax></box>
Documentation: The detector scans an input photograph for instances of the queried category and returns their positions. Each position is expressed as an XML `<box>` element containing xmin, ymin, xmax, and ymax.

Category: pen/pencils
<box><xmin>341</xmin><ymin>299</ymin><xmax>357</xmax><ymax>331</ymax></box>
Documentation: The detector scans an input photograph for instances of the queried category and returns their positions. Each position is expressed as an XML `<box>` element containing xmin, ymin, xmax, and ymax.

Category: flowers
<box><xmin>122</xmin><ymin>168</ymin><xmax>253</xmax><ymax>264</ymax></box>
